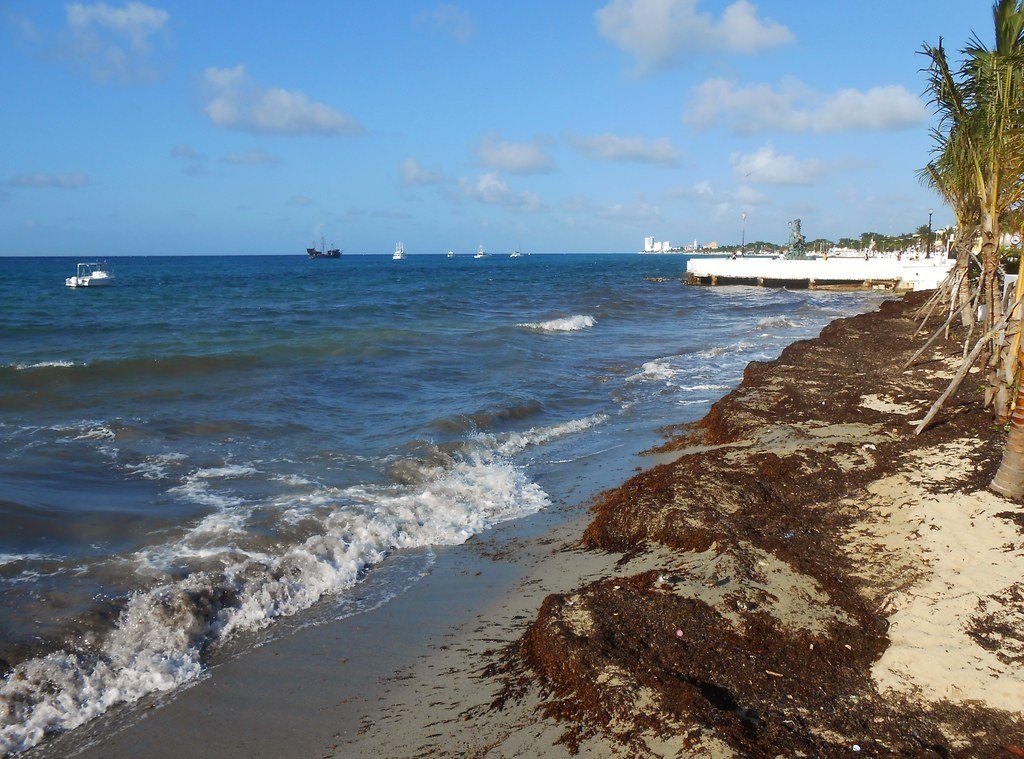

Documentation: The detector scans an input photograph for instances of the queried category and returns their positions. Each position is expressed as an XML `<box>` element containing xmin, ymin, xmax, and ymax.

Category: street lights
<box><xmin>788</xmin><ymin>221</ymin><xmax>792</xmax><ymax>244</ymax></box>
<box><xmin>741</xmin><ymin>213</ymin><xmax>747</xmax><ymax>256</ymax></box>
<box><xmin>925</xmin><ymin>209</ymin><xmax>933</xmax><ymax>258</ymax></box>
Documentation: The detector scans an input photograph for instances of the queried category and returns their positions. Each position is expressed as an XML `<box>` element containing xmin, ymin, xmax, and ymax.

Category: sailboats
<box><xmin>474</xmin><ymin>246</ymin><xmax>486</xmax><ymax>258</ymax></box>
<box><xmin>307</xmin><ymin>236</ymin><xmax>341</xmax><ymax>258</ymax></box>
<box><xmin>393</xmin><ymin>241</ymin><xmax>407</xmax><ymax>259</ymax></box>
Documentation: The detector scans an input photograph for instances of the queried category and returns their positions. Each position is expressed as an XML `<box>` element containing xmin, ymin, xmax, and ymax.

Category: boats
<box><xmin>66</xmin><ymin>263</ymin><xmax>112</xmax><ymax>286</ymax></box>
<box><xmin>510</xmin><ymin>252</ymin><xmax>521</xmax><ymax>258</ymax></box>
<box><xmin>448</xmin><ymin>251</ymin><xmax>454</xmax><ymax>257</ymax></box>
